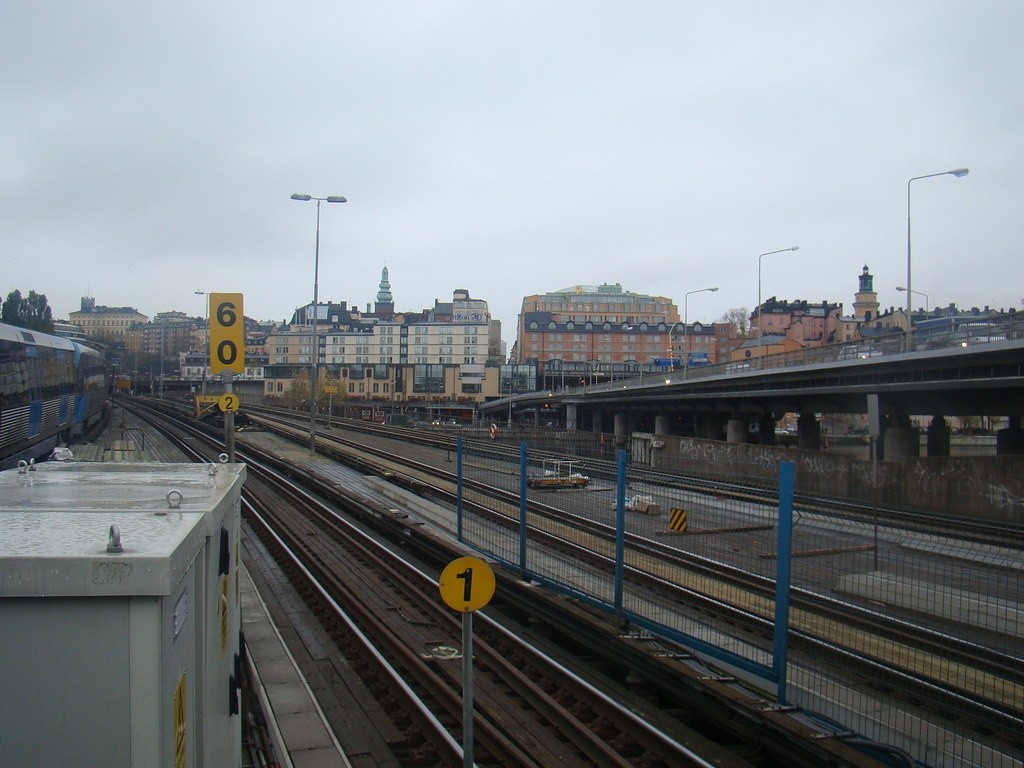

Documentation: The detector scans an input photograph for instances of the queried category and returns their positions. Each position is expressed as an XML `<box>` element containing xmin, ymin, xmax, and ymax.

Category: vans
<box><xmin>957</xmin><ymin>322</ymin><xmax>1006</xmax><ymax>345</ymax></box>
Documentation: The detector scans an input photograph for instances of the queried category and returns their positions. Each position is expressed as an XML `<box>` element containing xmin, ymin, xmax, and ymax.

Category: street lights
<box><xmin>896</xmin><ymin>285</ymin><xmax>931</xmax><ymax>350</ymax></box>
<box><xmin>129</xmin><ymin>334</ymin><xmax>145</xmax><ymax>397</ymax></box>
<box><xmin>195</xmin><ymin>290</ymin><xmax>211</xmax><ymax>409</ymax></box>
<box><xmin>905</xmin><ymin>167</ymin><xmax>971</xmax><ymax>353</ymax></box>
<box><xmin>609</xmin><ymin>326</ymin><xmax>634</xmax><ymax>390</ymax></box>
<box><xmin>505</xmin><ymin>379</ymin><xmax>512</xmax><ymax>429</ymax></box>
<box><xmin>589</xmin><ymin>337</ymin><xmax>609</xmax><ymax>387</ymax></box>
<box><xmin>638</xmin><ymin>310</ymin><xmax>668</xmax><ymax>385</ymax></box>
<box><xmin>683</xmin><ymin>286</ymin><xmax>719</xmax><ymax>379</ymax></box>
<box><xmin>325</xmin><ymin>378</ymin><xmax>336</xmax><ymax>429</ymax></box>
<box><xmin>390</xmin><ymin>378</ymin><xmax>398</xmax><ymax>415</ymax></box>
<box><xmin>668</xmin><ymin>322</ymin><xmax>678</xmax><ymax>370</ymax></box>
<box><xmin>154</xmin><ymin>317</ymin><xmax>173</xmax><ymax>400</ymax></box>
<box><xmin>756</xmin><ymin>245</ymin><xmax>801</xmax><ymax>370</ymax></box>
<box><xmin>289</xmin><ymin>192</ymin><xmax>348</xmax><ymax>456</ymax></box>
<box><xmin>561</xmin><ymin>338</ymin><xmax>580</xmax><ymax>391</ymax></box>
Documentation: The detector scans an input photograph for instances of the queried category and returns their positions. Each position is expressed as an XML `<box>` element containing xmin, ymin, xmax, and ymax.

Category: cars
<box><xmin>775</xmin><ymin>428</ymin><xmax>790</xmax><ymax>437</ymax></box>
<box><xmin>925</xmin><ymin>330</ymin><xmax>968</xmax><ymax>349</ymax></box>
<box><xmin>837</xmin><ymin>344</ymin><xmax>884</xmax><ymax>362</ymax></box>
<box><xmin>724</xmin><ymin>361</ymin><xmax>750</xmax><ymax>375</ymax></box>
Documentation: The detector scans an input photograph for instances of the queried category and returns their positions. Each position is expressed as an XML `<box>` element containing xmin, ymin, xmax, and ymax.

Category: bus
<box><xmin>375</xmin><ymin>410</ymin><xmax>385</xmax><ymax>423</ymax></box>
<box><xmin>361</xmin><ymin>409</ymin><xmax>372</xmax><ymax>421</ymax></box>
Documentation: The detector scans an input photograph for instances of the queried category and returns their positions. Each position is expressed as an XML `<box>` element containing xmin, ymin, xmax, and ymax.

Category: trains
<box><xmin>0</xmin><ymin>320</ymin><xmax>109</xmax><ymax>471</ymax></box>
